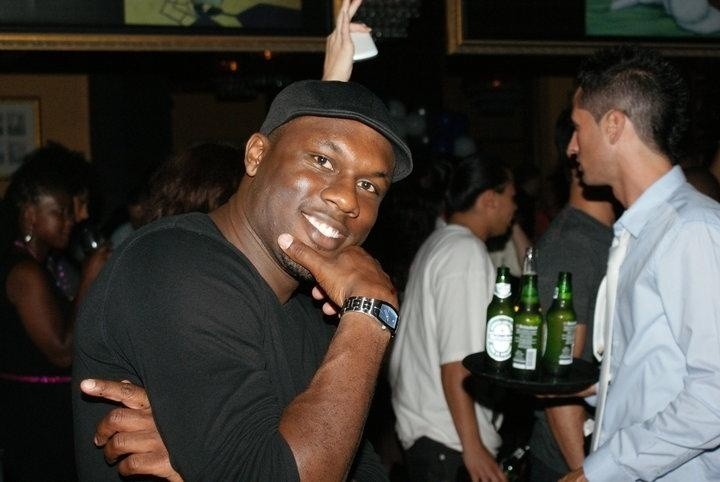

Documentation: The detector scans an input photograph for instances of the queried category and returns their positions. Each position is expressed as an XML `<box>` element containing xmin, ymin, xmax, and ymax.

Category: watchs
<box><xmin>343</xmin><ymin>294</ymin><xmax>400</xmax><ymax>335</ymax></box>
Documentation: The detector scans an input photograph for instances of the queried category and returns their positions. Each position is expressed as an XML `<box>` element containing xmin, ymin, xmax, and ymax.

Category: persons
<box><xmin>1</xmin><ymin>130</ymin><xmax>243</xmax><ymax>482</ymax></box>
<box><xmin>525</xmin><ymin>112</ymin><xmax>615</xmax><ymax>482</ymax></box>
<box><xmin>391</xmin><ymin>142</ymin><xmax>532</xmax><ymax>482</ymax></box>
<box><xmin>71</xmin><ymin>79</ymin><xmax>413</xmax><ymax>482</ymax></box>
<box><xmin>318</xmin><ymin>0</ymin><xmax>377</xmax><ymax>82</ymax></box>
<box><xmin>567</xmin><ymin>59</ymin><xmax>720</xmax><ymax>482</ymax></box>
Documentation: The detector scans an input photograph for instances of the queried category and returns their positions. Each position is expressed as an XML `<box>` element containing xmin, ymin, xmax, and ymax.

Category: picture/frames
<box><xmin>0</xmin><ymin>0</ymin><xmax>344</xmax><ymax>52</ymax></box>
<box><xmin>0</xmin><ymin>94</ymin><xmax>42</xmax><ymax>182</ymax></box>
<box><xmin>445</xmin><ymin>0</ymin><xmax>720</xmax><ymax>58</ymax></box>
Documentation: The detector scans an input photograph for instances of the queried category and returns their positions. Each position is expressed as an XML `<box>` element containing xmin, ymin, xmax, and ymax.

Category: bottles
<box><xmin>500</xmin><ymin>444</ymin><xmax>531</xmax><ymax>481</ymax></box>
<box><xmin>484</xmin><ymin>247</ymin><xmax>577</xmax><ymax>380</ymax></box>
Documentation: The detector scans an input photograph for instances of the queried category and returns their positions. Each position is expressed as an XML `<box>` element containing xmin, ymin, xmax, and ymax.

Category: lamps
<box><xmin>351</xmin><ymin>1</ymin><xmax>422</xmax><ymax>39</ymax></box>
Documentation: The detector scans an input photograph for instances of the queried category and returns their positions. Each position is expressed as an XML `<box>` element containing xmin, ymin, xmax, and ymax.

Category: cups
<box><xmin>80</xmin><ymin>223</ymin><xmax>106</xmax><ymax>256</ymax></box>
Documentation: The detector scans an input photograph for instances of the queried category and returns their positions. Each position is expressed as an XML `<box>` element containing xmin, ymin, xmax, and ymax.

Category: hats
<box><xmin>261</xmin><ymin>80</ymin><xmax>413</xmax><ymax>183</ymax></box>
